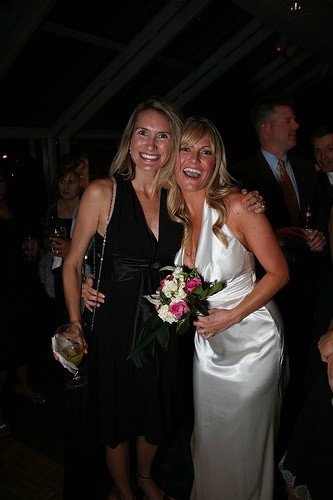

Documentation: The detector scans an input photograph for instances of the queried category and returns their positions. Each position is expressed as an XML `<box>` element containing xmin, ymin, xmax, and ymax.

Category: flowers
<box><xmin>143</xmin><ymin>266</ymin><xmax>226</xmax><ymax>334</ymax></box>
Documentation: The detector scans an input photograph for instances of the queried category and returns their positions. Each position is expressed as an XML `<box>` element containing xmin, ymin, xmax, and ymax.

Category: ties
<box><xmin>278</xmin><ymin>159</ymin><xmax>301</xmax><ymax>221</ymax></box>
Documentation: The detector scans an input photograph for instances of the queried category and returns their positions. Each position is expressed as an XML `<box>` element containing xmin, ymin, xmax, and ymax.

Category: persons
<box><xmin>80</xmin><ymin>116</ymin><xmax>291</xmax><ymax>500</ymax></box>
<box><xmin>229</xmin><ymin>99</ymin><xmax>333</xmax><ymax>499</ymax></box>
<box><xmin>0</xmin><ymin>153</ymin><xmax>108</xmax><ymax>447</ymax></box>
<box><xmin>64</xmin><ymin>100</ymin><xmax>266</xmax><ymax>500</ymax></box>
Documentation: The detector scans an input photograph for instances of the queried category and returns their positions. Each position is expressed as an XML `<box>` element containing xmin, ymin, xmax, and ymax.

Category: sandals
<box><xmin>0</xmin><ymin>421</ymin><xmax>11</xmax><ymax>438</ymax></box>
<box><xmin>15</xmin><ymin>382</ymin><xmax>48</xmax><ymax>409</ymax></box>
<box><xmin>137</xmin><ymin>470</ymin><xmax>171</xmax><ymax>500</ymax></box>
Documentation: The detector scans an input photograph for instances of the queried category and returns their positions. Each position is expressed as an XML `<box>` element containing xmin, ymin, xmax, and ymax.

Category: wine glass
<box><xmin>49</xmin><ymin>226</ymin><xmax>67</xmax><ymax>256</ymax></box>
<box><xmin>55</xmin><ymin>324</ymin><xmax>89</xmax><ymax>390</ymax></box>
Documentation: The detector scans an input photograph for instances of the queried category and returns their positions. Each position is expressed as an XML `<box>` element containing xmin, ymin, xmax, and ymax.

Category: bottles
<box><xmin>302</xmin><ymin>202</ymin><xmax>314</xmax><ymax>252</ymax></box>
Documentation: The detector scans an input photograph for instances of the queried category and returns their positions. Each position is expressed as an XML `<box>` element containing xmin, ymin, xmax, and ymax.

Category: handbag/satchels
<box><xmin>63</xmin><ymin>317</ymin><xmax>97</xmax><ymax>392</ymax></box>
